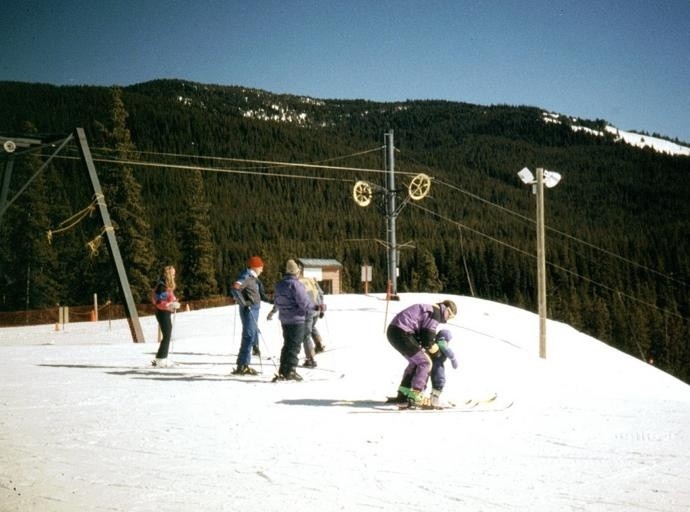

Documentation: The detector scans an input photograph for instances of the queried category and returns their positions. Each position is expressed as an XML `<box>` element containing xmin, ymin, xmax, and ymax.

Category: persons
<box><xmin>151</xmin><ymin>265</ymin><xmax>180</xmax><ymax>369</ymax></box>
<box><xmin>387</xmin><ymin>300</ymin><xmax>457</xmax><ymax>408</ymax></box>
<box><xmin>230</xmin><ymin>257</ymin><xmax>327</xmax><ymax>380</ymax></box>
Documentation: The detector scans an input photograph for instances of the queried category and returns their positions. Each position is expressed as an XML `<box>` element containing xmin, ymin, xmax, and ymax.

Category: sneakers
<box><xmin>303</xmin><ymin>357</ymin><xmax>317</xmax><ymax>367</ymax></box>
<box><xmin>154</xmin><ymin>356</ymin><xmax>174</xmax><ymax>368</ymax></box>
<box><xmin>399</xmin><ymin>386</ymin><xmax>411</xmax><ymax>401</ymax></box>
<box><xmin>237</xmin><ymin>364</ymin><xmax>258</xmax><ymax>376</ymax></box>
<box><xmin>430</xmin><ymin>389</ymin><xmax>444</xmax><ymax>410</ymax></box>
<box><xmin>253</xmin><ymin>345</ymin><xmax>262</xmax><ymax>355</ymax></box>
<box><xmin>407</xmin><ymin>389</ymin><xmax>427</xmax><ymax>406</ymax></box>
<box><xmin>278</xmin><ymin>365</ymin><xmax>303</xmax><ymax>380</ymax></box>
<box><xmin>314</xmin><ymin>341</ymin><xmax>326</xmax><ymax>354</ymax></box>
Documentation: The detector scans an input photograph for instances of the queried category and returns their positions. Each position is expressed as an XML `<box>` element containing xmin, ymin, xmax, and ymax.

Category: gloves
<box><xmin>434</xmin><ymin>351</ymin><xmax>448</xmax><ymax>363</ymax></box>
<box><xmin>321</xmin><ymin>305</ymin><xmax>327</xmax><ymax>319</ymax></box>
<box><xmin>267</xmin><ymin>311</ymin><xmax>275</xmax><ymax>320</ymax></box>
<box><xmin>451</xmin><ymin>360</ymin><xmax>458</xmax><ymax>369</ymax></box>
<box><xmin>243</xmin><ymin>304</ymin><xmax>252</xmax><ymax>314</ymax></box>
<box><xmin>312</xmin><ymin>304</ymin><xmax>321</xmax><ymax>312</ymax></box>
<box><xmin>167</xmin><ymin>302</ymin><xmax>181</xmax><ymax>310</ymax></box>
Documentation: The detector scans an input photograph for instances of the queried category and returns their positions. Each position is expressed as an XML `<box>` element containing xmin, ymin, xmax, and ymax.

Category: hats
<box><xmin>246</xmin><ymin>256</ymin><xmax>264</xmax><ymax>268</ymax></box>
<box><xmin>285</xmin><ymin>259</ymin><xmax>300</xmax><ymax>276</ymax></box>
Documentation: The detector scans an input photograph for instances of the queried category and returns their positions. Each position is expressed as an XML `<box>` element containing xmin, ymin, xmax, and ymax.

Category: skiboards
<box><xmin>219</xmin><ymin>346</ymin><xmax>345</xmax><ymax>381</ymax></box>
<box><xmin>126</xmin><ymin>364</ymin><xmax>197</xmax><ymax>371</ymax></box>
<box><xmin>333</xmin><ymin>394</ymin><xmax>514</xmax><ymax>412</ymax></box>
